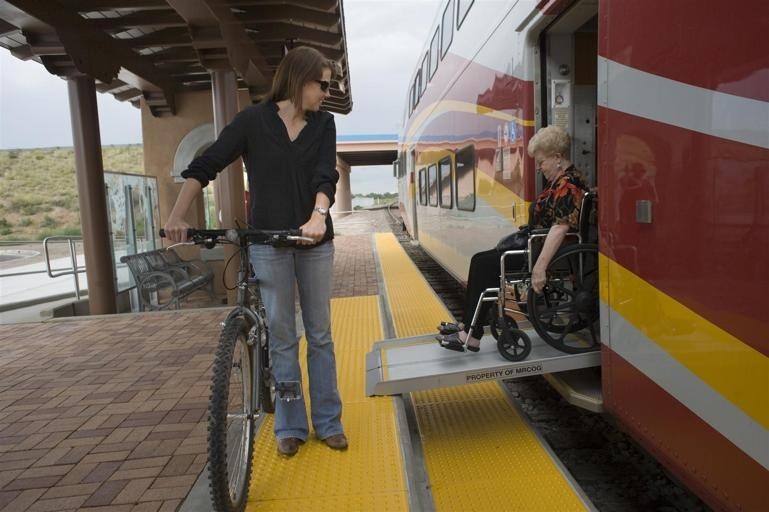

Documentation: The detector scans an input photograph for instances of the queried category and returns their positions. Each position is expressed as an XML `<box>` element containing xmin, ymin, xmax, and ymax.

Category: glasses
<box><xmin>315</xmin><ymin>80</ymin><xmax>329</xmax><ymax>92</ymax></box>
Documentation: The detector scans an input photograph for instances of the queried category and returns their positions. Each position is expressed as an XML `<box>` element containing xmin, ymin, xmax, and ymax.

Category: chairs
<box><xmin>119</xmin><ymin>246</ymin><xmax>215</xmax><ymax>310</ymax></box>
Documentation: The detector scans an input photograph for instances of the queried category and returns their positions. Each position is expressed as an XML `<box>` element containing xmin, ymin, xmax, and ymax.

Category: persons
<box><xmin>429</xmin><ymin>125</ymin><xmax>592</xmax><ymax>352</ymax></box>
<box><xmin>168</xmin><ymin>43</ymin><xmax>348</xmax><ymax>455</ymax></box>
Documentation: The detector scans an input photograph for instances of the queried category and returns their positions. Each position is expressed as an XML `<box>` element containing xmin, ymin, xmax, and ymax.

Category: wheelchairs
<box><xmin>440</xmin><ymin>187</ymin><xmax>599</xmax><ymax>363</ymax></box>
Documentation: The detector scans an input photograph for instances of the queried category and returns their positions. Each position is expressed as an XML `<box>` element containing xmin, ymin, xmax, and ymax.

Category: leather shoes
<box><xmin>278</xmin><ymin>437</ymin><xmax>297</xmax><ymax>454</ymax></box>
<box><xmin>326</xmin><ymin>434</ymin><xmax>347</xmax><ymax>448</ymax></box>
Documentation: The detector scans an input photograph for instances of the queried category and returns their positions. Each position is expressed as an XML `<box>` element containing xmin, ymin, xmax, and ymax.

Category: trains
<box><xmin>362</xmin><ymin>1</ymin><xmax>769</xmax><ymax>504</ymax></box>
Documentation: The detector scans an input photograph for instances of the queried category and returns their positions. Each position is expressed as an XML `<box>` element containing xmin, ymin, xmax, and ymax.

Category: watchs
<box><xmin>309</xmin><ymin>207</ymin><xmax>327</xmax><ymax>217</ymax></box>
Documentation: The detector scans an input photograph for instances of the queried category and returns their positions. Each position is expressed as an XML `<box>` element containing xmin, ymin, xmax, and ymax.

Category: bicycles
<box><xmin>158</xmin><ymin>225</ymin><xmax>316</xmax><ymax>512</ymax></box>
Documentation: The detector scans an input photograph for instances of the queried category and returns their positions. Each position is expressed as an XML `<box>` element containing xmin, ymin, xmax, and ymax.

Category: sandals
<box><xmin>435</xmin><ymin>324</ymin><xmax>480</xmax><ymax>352</ymax></box>
